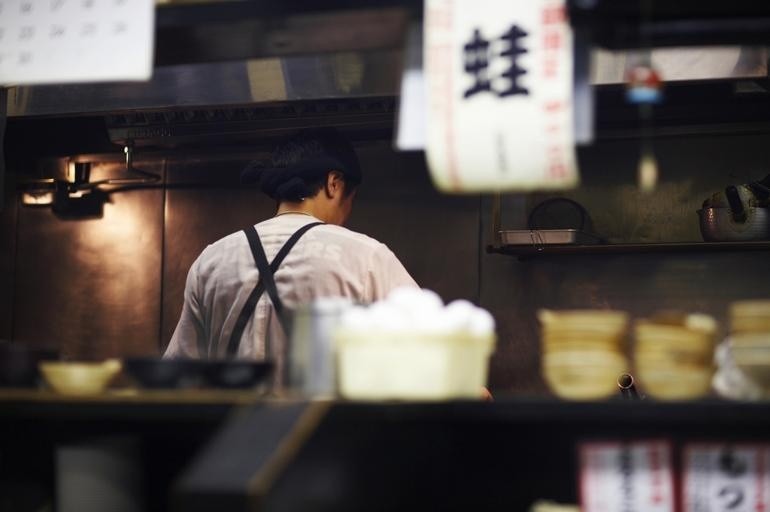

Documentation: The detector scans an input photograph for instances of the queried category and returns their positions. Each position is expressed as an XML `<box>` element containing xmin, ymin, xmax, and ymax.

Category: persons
<box><xmin>162</xmin><ymin>131</ymin><xmax>422</xmax><ymax>392</ymax></box>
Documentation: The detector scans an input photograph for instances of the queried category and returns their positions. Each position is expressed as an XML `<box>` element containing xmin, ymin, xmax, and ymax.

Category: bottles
<box><xmin>284</xmin><ymin>300</ymin><xmax>344</xmax><ymax>401</ymax></box>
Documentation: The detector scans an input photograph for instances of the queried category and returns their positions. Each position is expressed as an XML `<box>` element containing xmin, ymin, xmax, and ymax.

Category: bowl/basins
<box><xmin>535</xmin><ymin>303</ymin><xmax>769</xmax><ymax>401</ymax></box>
<box><xmin>331</xmin><ymin>332</ymin><xmax>498</xmax><ymax>401</ymax></box>
<box><xmin>37</xmin><ymin>355</ymin><xmax>279</xmax><ymax>394</ymax></box>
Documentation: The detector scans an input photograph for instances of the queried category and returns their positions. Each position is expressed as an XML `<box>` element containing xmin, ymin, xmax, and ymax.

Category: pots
<box><xmin>695</xmin><ymin>182</ymin><xmax>770</xmax><ymax>243</ymax></box>
